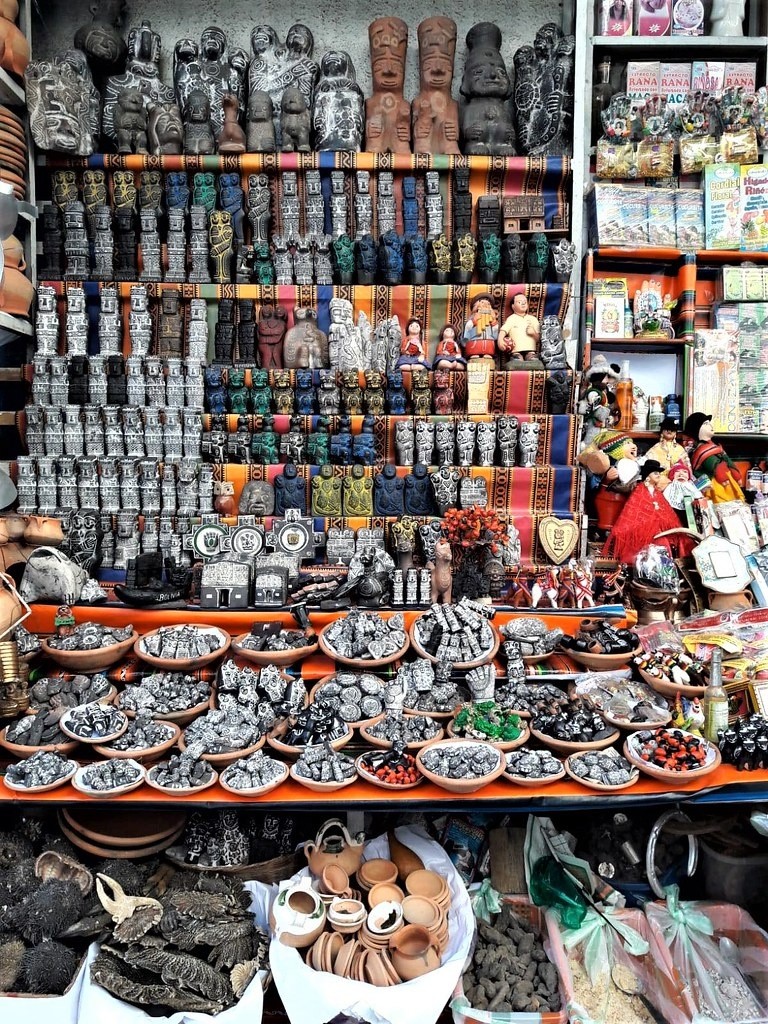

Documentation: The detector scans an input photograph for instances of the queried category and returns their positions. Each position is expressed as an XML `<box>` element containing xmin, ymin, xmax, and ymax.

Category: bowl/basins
<box><xmin>308</xmin><ymin>857</ymin><xmax>453</xmax><ymax>987</ymax></box>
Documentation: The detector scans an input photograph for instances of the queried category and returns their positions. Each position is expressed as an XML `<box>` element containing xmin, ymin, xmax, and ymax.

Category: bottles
<box><xmin>702</xmin><ymin>647</ymin><xmax>731</xmax><ymax>748</ymax></box>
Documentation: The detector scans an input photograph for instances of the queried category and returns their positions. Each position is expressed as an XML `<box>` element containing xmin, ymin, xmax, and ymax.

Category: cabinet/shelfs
<box><xmin>2</xmin><ymin>0</ymin><xmax>766</xmax><ymax>806</ymax></box>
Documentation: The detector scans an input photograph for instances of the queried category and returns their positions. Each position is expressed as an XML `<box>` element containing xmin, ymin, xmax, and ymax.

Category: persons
<box><xmin>14</xmin><ymin>0</ymin><xmax>768</xmax><ymax>702</ymax></box>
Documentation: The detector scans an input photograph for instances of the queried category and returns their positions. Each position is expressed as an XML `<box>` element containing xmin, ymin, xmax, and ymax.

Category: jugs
<box><xmin>268</xmin><ymin>876</ymin><xmax>327</xmax><ymax>949</ymax></box>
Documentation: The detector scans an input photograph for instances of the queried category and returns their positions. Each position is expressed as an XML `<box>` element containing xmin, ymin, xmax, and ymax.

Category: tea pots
<box><xmin>303</xmin><ymin>819</ymin><xmax>365</xmax><ymax>881</ymax></box>
